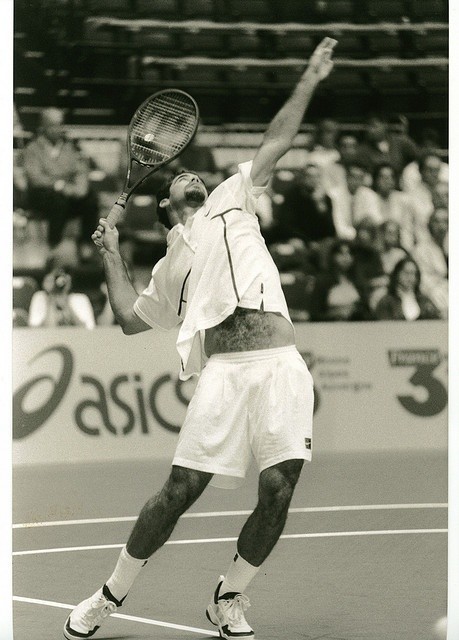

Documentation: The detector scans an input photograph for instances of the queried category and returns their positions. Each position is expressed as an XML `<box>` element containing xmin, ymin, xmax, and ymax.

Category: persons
<box><xmin>23</xmin><ymin>107</ymin><xmax>98</xmax><ymax>255</ymax></box>
<box><xmin>175</xmin><ymin>114</ymin><xmax>224</xmax><ymax>195</ymax></box>
<box><xmin>270</xmin><ymin>113</ymin><xmax>449</xmax><ymax>321</ymax></box>
<box><xmin>62</xmin><ymin>36</ymin><xmax>339</xmax><ymax>640</ymax></box>
<box><xmin>124</xmin><ymin>115</ymin><xmax>179</xmax><ymax>230</ymax></box>
<box><xmin>27</xmin><ymin>247</ymin><xmax>97</xmax><ymax>328</ymax></box>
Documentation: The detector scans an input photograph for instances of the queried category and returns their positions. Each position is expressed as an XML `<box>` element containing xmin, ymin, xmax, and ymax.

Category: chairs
<box><xmin>179</xmin><ymin>145</ymin><xmax>224</xmax><ymax>173</ymax></box>
<box><xmin>126</xmin><ymin>196</ymin><xmax>170</xmax><ymax>265</ymax></box>
<box><xmin>56</xmin><ymin>1</ymin><xmax>449</xmax><ymax>125</ymax></box>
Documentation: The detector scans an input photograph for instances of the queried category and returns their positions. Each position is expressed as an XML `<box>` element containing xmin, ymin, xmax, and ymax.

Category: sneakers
<box><xmin>206</xmin><ymin>575</ymin><xmax>254</xmax><ymax>640</ymax></box>
<box><xmin>63</xmin><ymin>583</ymin><xmax>127</xmax><ymax>640</ymax></box>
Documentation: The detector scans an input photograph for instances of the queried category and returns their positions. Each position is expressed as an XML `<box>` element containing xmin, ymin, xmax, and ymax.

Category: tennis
<box><xmin>434</xmin><ymin>617</ymin><xmax>447</xmax><ymax>639</ymax></box>
